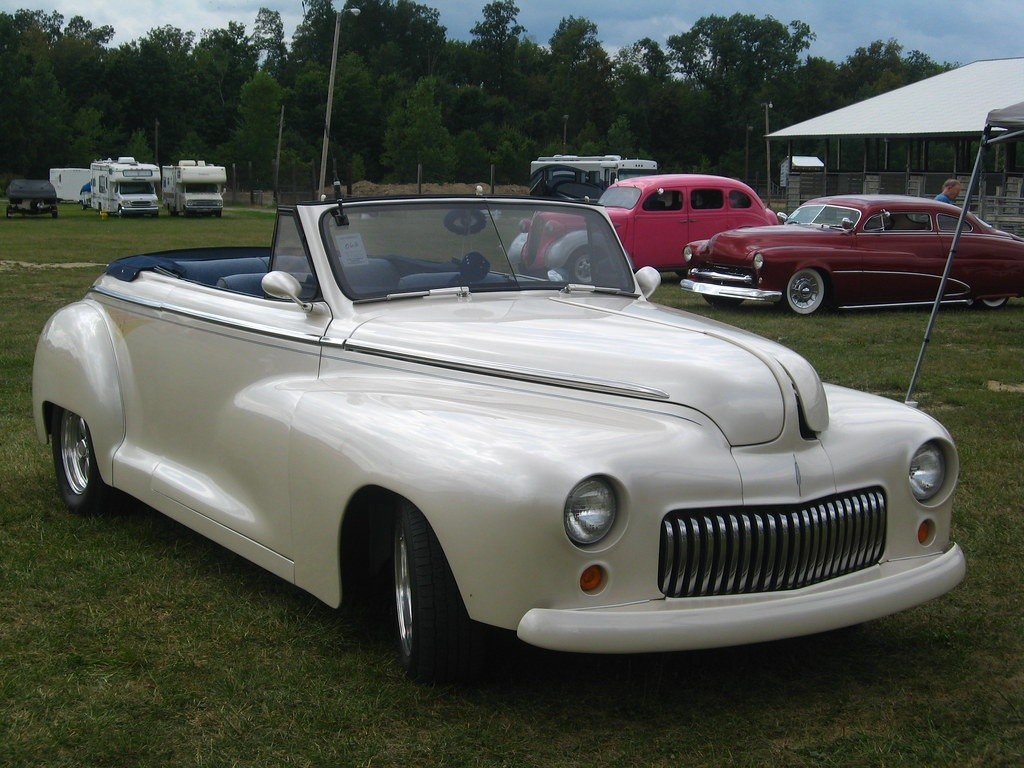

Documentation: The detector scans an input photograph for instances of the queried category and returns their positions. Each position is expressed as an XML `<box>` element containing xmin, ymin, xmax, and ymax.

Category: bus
<box><xmin>529</xmin><ymin>153</ymin><xmax>659</xmax><ymax>205</ymax></box>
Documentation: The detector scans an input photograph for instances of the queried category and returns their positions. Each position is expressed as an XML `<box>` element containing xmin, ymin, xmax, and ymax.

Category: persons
<box><xmin>935</xmin><ymin>179</ymin><xmax>962</xmax><ymax>206</ymax></box>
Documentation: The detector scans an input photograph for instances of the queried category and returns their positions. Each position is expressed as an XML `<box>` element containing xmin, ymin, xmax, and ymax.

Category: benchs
<box><xmin>175</xmin><ymin>256</ymin><xmax>403</xmax><ymax>287</ymax></box>
<box><xmin>926</xmin><ymin>219</ymin><xmax>969</xmax><ymax>230</ymax></box>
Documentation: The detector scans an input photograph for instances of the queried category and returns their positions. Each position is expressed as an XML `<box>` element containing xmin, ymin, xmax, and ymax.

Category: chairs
<box><xmin>397</xmin><ymin>271</ymin><xmax>508</xmax><ymax>288</ymax></box>
<box><xmin>648</xmin><ymin>200</ymin><xmax>665</xmax><ymax>210</ymax></box>
<box><xmin>871</xmin><ymin>215</ymin><xmax>894</xmax><ymax>229</ymax></box>
<box><xmin>216</xmin><ymin>272</ymin><xmax>322</xmax><ymax>300</ymax></box>
<box><xmin>670</xmin><ymin>200</ymin><xmax>696</xmax><ymax>210</ymax></box>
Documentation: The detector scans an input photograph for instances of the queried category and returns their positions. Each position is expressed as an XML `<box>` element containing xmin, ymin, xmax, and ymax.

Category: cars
<box><xmin>504</xmin><ymin>172</ymin><xmax>781</xmax><ymax>285</ymax></box>
<box><xmin>4</xmin><ymin>180</ymin><xmax>58</xmax><ymax>219</ymax></box>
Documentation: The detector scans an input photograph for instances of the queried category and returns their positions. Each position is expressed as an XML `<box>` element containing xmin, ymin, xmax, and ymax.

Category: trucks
<box><xmin>161</xmin><ymin>159</ymin><xmax>227</xmax><ymax>218</ymax></box>
<box><xmin>88</xmin><ymin>156</ymin><xmax>162</xmax><ymax>219</ymax></box>
<box><xmin>48</xmin><ymin>167</ymin><xmax>92</xmax><ymax>211</ymax></box>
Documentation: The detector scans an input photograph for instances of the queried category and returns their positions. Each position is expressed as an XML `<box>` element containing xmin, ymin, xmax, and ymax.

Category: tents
<box><xmin>903</xmin><ymin>101</ymin><xmax>1024</xmax><ymax>411</ymax></box>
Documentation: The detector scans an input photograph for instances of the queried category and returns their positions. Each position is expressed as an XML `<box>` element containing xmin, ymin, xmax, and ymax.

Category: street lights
<box><xmin>744</xmin><ymin>122</ymin><xmax>754</xmax><ymax>184</ymax></box>
<box><xmin>317</xmin><ymin>6</ymin><xmax>362</xmax><ymax>201</ymax></box>
<box><xmin>759</xmin><ymin>101</ymin><xmax>775</xmax><ymax>210</ymax></box>
<box><xmin>562</xmin><ymin>114</ymin><xmax>570</xmax><ymax>156</ymax></box>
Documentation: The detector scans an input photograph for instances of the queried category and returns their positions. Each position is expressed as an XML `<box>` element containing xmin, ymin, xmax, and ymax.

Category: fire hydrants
<box><xmin>101</xmin><ymin>207</ymin><xmax>109</xmax><ymax>221</ymax></box>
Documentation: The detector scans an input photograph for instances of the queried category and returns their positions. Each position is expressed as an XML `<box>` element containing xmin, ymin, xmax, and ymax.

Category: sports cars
<box><xmin>27</xmin><ymin>186</ymin><xmax>967</xmax><ymax>686</ymax></box>
<box><xmin>677</xmin><ymin>192</ymin><xmax>1024</xmax><ymax>316</ymax></box>
